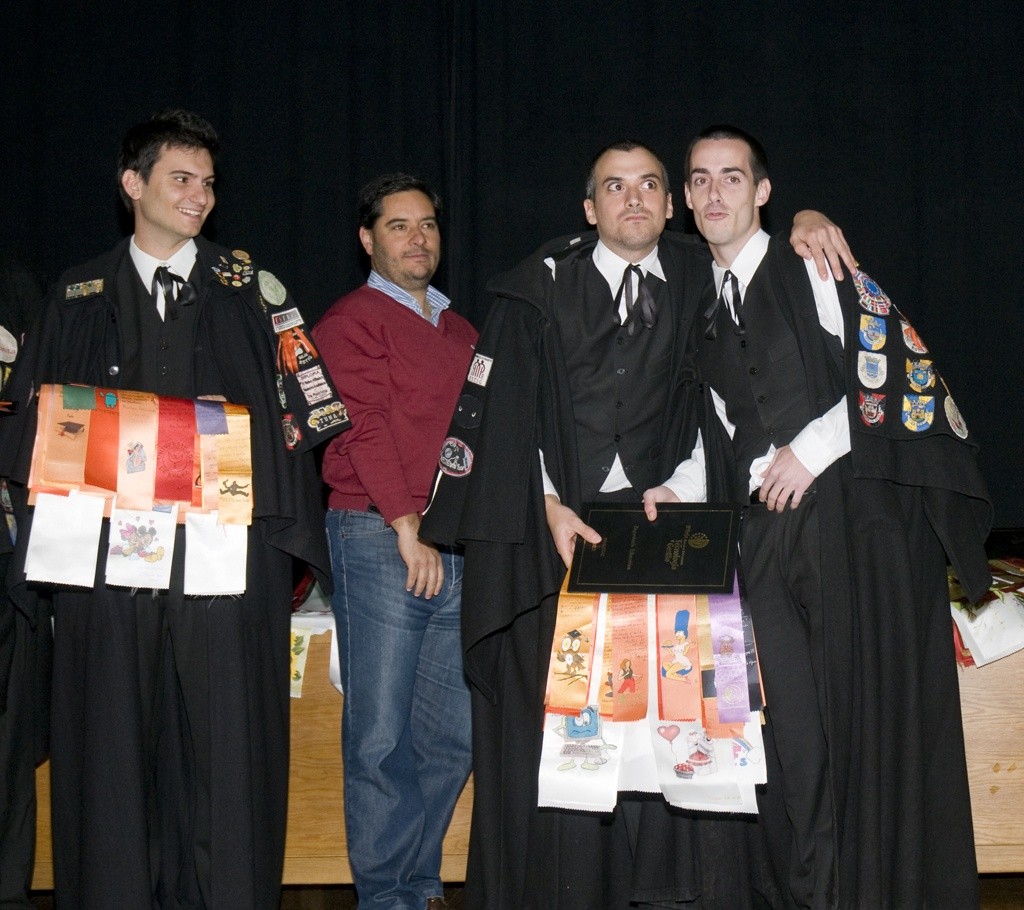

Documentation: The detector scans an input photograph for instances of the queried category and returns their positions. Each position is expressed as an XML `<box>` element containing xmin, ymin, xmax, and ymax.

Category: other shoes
<box><xmin>427</xmin><ymin>897</ymin><xmax>450</xmax><ymax>910</ymax></box>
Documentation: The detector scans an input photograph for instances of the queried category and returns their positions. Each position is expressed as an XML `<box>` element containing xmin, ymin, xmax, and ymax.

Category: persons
<box><xmin>420</xmin><ymin>145</ymin><xmax>863</xmax><ymax>909</ymax></box>
<box><xmin>1</xmin><ymin>109</ymin><xmax>321</xmax><ymax>910</ymax></box>
<box><xmin>642</xmin><ymin>125</ymin><xmax>995</xmax><ymax>910</ymax></box>
<box><xmin>314</xmin><ymin>174</ymin><xmax>474</xmax><ymax>909</ymax></box>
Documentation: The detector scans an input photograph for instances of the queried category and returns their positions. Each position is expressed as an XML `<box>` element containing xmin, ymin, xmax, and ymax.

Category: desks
<box><xmin>34</xmin><ymin>575</ymin><xmax>1024</xmax><ymax>889</ymax></box>
